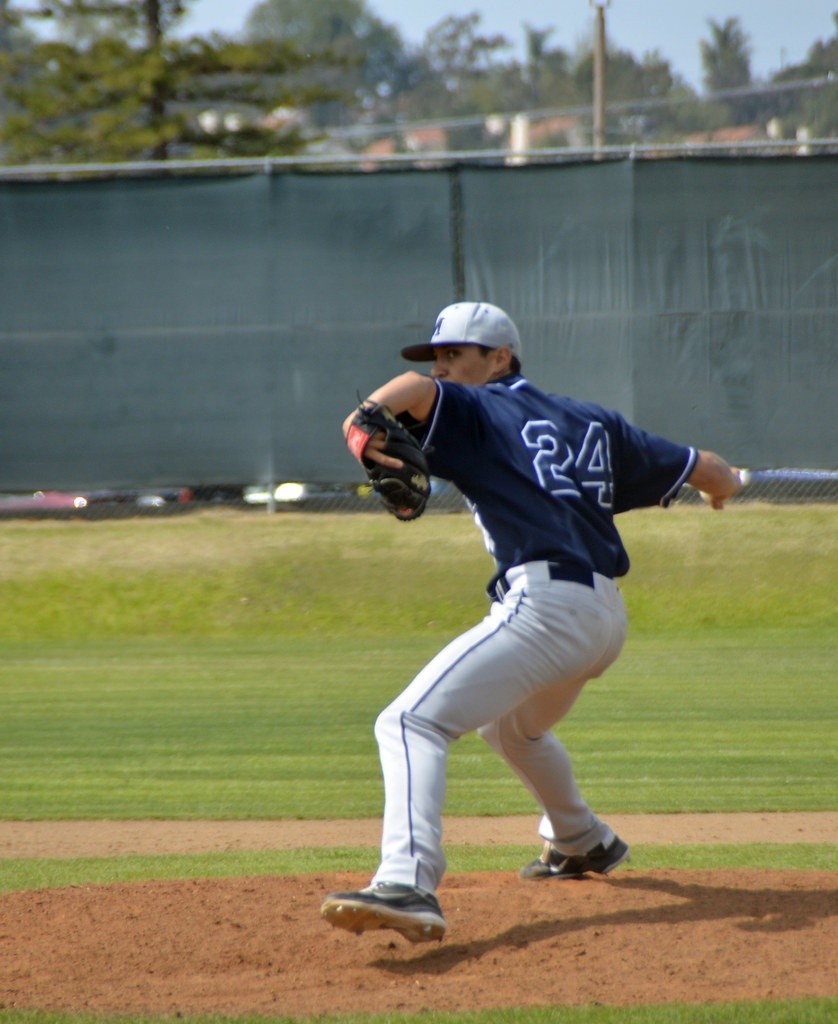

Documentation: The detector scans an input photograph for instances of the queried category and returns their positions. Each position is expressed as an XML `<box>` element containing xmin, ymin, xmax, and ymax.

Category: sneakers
<box><xmin>319</xmin><ymin>883</ymin><xmax>445</xmax><ymax>943</ymax></box>
<box><xmin>520</xmin><ymin>825</ymin><xmax>629</xmax><ymax>881</ymax></box>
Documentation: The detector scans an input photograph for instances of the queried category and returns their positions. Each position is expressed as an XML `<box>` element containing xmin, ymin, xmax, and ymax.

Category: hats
<box><xmin>401</xmin><ymin>302</ymin><xmax>522</xmax><ymax>364</ymax></box>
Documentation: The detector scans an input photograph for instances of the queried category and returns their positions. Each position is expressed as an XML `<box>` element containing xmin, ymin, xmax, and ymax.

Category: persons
<box><xmin>317</xmin><ymin>299</ymin><xmax>746</xmax><ymax>943</ymax></box>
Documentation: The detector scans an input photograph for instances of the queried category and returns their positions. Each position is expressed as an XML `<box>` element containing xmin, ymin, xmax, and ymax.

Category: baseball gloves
<box><xmin>344</xmin><ymin>401</ymin><xmax>432</xmax><ymax>522</ymax></box>
